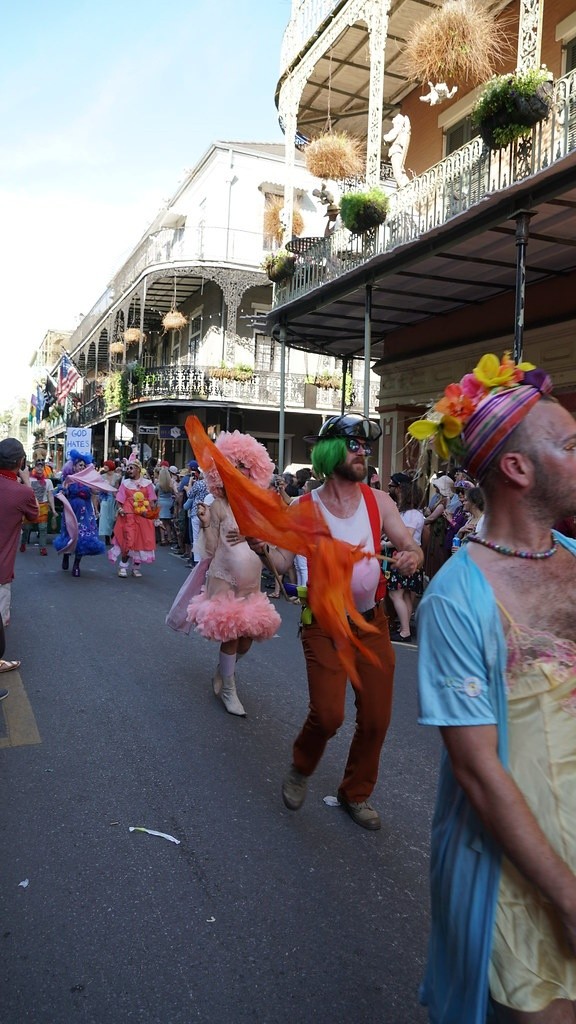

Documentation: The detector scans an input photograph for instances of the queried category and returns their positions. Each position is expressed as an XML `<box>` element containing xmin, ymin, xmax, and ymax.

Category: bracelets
<box><xmin>155</xmin><ymin>520</ymin><xmax>163</xmax><ymax>526</ymax></box>
<box><xmin>118</xmin><ymin>508</ymin><xmax>123</xmax><ymax>512</ymax></box>
<box><xmin>203</xmin><ymin>526</ymin><xmax>209</xmax><ymax>528</ymax></box>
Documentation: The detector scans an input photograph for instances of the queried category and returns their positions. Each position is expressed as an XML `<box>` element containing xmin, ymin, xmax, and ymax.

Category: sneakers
<box><xmin>338</xmin><ymin>794</ymin><xmax>381</xmax><ymax>830</ymax></box>
<box><xmin>282</xmin><ymin>764</ymin><xmax>307</xmax><ymax>810</ymax></box>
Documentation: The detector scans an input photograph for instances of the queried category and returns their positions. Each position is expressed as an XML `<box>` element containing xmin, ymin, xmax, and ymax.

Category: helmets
<box><xmin>304</xmin><ymin>413</ymin><xmax>383</xmax><ymax>440</ymax></box>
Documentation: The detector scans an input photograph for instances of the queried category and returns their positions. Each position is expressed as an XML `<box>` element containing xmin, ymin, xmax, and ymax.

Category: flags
<box><xmin>28</xmin><ymin>355</ymin><xmax>80</xmax><ymax>424</ymax></box>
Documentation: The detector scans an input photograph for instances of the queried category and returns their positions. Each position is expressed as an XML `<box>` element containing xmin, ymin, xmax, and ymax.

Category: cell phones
<box><xmin>21</xmin><ymin>459</ymin><xmax>26</xmax><ymax>470</ymax></box>
<box><xmin>191</xmin><ymin>471</ymin><xmax>196</xmax><ymax>481</ymax></box>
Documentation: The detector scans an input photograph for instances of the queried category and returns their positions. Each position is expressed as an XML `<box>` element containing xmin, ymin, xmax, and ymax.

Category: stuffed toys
<box><xmin>132</xmin><ymin>492</ymin><xmax>149</xmax><ymax>513</ymax></box>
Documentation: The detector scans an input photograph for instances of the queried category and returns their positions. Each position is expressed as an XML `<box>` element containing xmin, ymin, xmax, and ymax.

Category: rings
<box><xmin>234</xmin><ymin>537</ymin><xmax>236</xmax><ymax>542</ymax></box>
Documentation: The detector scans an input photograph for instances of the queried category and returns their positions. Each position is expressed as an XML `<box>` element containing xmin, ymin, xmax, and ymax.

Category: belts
<box><xmin>312</xmin><ymin>602</ymin><xmax>380</xmax><ymax>626</ymax></box>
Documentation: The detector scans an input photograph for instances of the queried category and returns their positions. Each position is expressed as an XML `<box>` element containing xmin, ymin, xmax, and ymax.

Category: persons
<box><xmin>51</xmin><ymin>449</ymin><xmax>119</xmax><ymax>577</ymax></box>
<box><xmin>260</xmin><ymin>467</ymin><xmax>326</xmax><ymax>604</ymax></box>
<box><xmin>187</xmin><ymin>429</ymin><xmax>282</xmax><ymax>715</ymax></box>
<box><xmin>366</xmin><ymin>466</ymin><xmax>485</xmax><ymax>643</ymax></box>
<box><xmin>245</xmin><ymin>411</ymin><xmax>424</xmax><ymax>830</ymax></box>
<box><xmin>93</xmin><ymin>456</ymin><xmax>126</xmax><ymax>545</ymax></box>
<box><xmin>140</xmin><ymin>457</ymin><xmax>215</xmax><ymax>593</ymax></box>
<box><xmin>18</xmin><ymin>448</ymin><xmax>64</xmax><ymax>556</ymax></box>
<box><xmin>106</xmin><ymin>459</ymin><xmax>167</xmax><ymax>577</ymax></box>
<box><xmin>383</xmin><ymin>113</ymin><xmax>410</xmax><ymax>187</ymax></box>
<box><xmin>410</xmin><ymin>352</ymin><xmax>576</xmax><ymax>1024</ymax></box>
<box><xmin>0</xmin><ymin>438</ymin><xmax>40</xmax><ymax>672</ymax></box>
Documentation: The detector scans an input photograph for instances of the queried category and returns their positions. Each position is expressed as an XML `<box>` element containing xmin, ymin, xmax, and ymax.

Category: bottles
<box><xmin>452</xmin><ymin>534</ymin><xmax>460</xmax><ymax>555</ymax></box>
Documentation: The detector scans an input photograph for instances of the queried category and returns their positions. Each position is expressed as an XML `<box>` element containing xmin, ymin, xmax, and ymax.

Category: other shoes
<box><xmin>20</xmin><ymin>543</ymin><xmax>26</xmax><ymax>552</ymax></box>
<box><xmin>0</xmin><ymin>689</ymin><xmax>9</xmax><ymax>700</ymax></box>
<box><xmin>219</xmin><ymin>677</ymin><xmax>248</xmax><ymax>716</ymax></box>
<box><xmin>60</xmin><ymin>539</ymin><xmax>196</xmax><ymax>580</ymax></box>
<box><xmin>391</xmin><ymin>633</ymin><xmax>412</xmax><ymax>642</ymax></box>
<box><xmin>41</xmin><ymin>547</ymin><xmax>47</xmax><ymax>555</ymax></box>
<box><xmin>0</xmin><ymin>660</ymin><xmax>21</xmax><ymax>673</ymax></box>
<box><xmin>212</xmin><ymin>666</ymin><xmax>223</xmax><ymax>695</ymax></box>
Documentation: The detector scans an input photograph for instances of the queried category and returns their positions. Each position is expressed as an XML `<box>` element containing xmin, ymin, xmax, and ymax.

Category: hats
<box><xmin>169</xmin><ymin>466</ymin><xmax>179</xmax><ymax>474</ymax></box>
<box><xmin>454</xmin><ymin>481</ymin><xmax>475</xmax><ymax>490</ymax></box>
<box><xmin>0</xmin><ymin>438</ymin><xmax>26</xmax><ymax>464</ymax></box>
<box><xmin>433</xmin><ymin>476</ymin><xmax>454</xmax><ymax>496</ymax></box>
<box><xmin>390</xmin><ymin>473</ymin><xmax>410</xmax><ymax>486</ymax></box>
<box><xmin>126</xmin><ymin>459</ymin><xmax>142</xmax><ymax>469</ymax></box>
<box><xmin>160</xmin><ymin>462</ymin><xmax>169</xmax><ymax>467</ymax></box>
<box><xmin>188</xmin><ymin>461</ymin><xmax>199</xmax><ymax>470</ymax></box>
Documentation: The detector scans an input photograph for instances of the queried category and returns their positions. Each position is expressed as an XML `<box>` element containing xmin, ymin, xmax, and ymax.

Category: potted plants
<box><xmin>118</xmin><ymin>360</ymin><xmax>144</xmax><ymax>417</ymax></box>
<box><xmin>234</xmin><ymin>362</ymin><xmax>256</xmax><ymax>381</ymax></box>
<box><xmin>307</xmin><ymin>371</ymin><xmax>357</xmax><ymax>405</ymax></box>
<box><xmin>468</xmin><ymin>70</ymin><xmax>554</xmax><ymax>152</ymax></box>
<box><xmin>339</xmin><ymin>188</ymin><xmax>389</xmax><ymax>231</ymax></box>
<box><xmin>264</xmin><ymin>256</ymin><xmax>295</xmax><ymax>282</ymax></box>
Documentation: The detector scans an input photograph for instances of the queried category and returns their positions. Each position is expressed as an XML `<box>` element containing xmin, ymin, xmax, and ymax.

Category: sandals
<box><xmin>268</xmin><ymin>593</ymin><xmax>281</xmax><ymax>599</ymax></box>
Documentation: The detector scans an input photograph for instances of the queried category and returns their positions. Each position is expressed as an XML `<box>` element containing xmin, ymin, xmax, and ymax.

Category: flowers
<box><xmin>408</xmin><ymin>351</ymin><xmax>534</xmax><ymax>458</ymax></box>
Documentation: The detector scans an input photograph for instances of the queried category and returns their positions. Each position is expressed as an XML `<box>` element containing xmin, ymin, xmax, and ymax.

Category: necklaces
<box><xmin>466</xmin><ymin>535</ymin><xmax>557</xmax><ymax>557</ymax></box>
<box><xmin>0</xmin><ymin>474</ymin><xmax>16</xmax><ymax>481</ymax></box>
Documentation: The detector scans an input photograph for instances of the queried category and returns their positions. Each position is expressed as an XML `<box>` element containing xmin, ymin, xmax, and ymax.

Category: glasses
<box><xmin>455</xmin><ymin>489</ymin><xmax>465</xmax><ymax>495</ymax></box>
<box><xmin>347</xmin><ymin>438</ymin><xmax>373</xmax><ymax>456</ymax></box>
<box><xmin>126</xmin><ymin>466</ymin><xmax>133</xmax><ymax>470</ymax></box>
